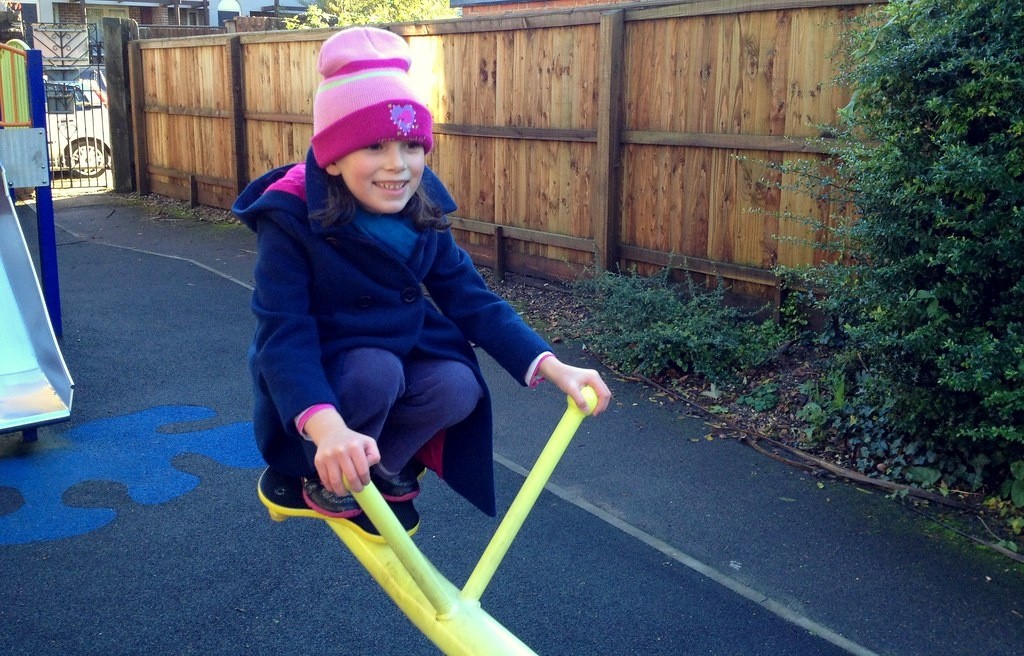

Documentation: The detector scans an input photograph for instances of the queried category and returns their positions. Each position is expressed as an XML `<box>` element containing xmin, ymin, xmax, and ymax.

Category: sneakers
<box><xmin>370</xmin><ymin>465</ymin><xmax>420</xmax><ymax>501</ymax></box>
<box><xmin>301</xmin><ymin>473</ymin><xmax>362</xmax><ymax>518</ymax></box>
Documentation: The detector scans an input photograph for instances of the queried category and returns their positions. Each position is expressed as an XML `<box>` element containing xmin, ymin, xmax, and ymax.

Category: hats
<box><xmin>311</xmin><ymin>27</ymin><xmax>433</xmax><ymax>169</ymax></box>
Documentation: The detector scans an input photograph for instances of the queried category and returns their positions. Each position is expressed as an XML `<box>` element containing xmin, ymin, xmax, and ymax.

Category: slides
<box><xmin>0</xmin><ymin>159</ymin><xmax>76</xmax><ymax>436</ymax></box>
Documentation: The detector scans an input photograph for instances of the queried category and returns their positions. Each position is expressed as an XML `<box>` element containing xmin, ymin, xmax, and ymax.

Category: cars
<box><xmin>44</xmin><ymin>79</ymin><xmax>109</xmax><ymax>178</ymax></box>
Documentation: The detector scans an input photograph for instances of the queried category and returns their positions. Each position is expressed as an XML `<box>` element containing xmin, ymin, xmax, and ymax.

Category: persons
<box><xmin>232</xmin><ymin>27</ymin><xmax>611</xmax><ymax>518</ymax></box>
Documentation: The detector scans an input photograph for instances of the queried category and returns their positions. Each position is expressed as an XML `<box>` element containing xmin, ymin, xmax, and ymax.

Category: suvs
<box><xmin>43</xmin><ymin>65</ymin><xmax>109</xmax><ymax>95</ymax></box>
